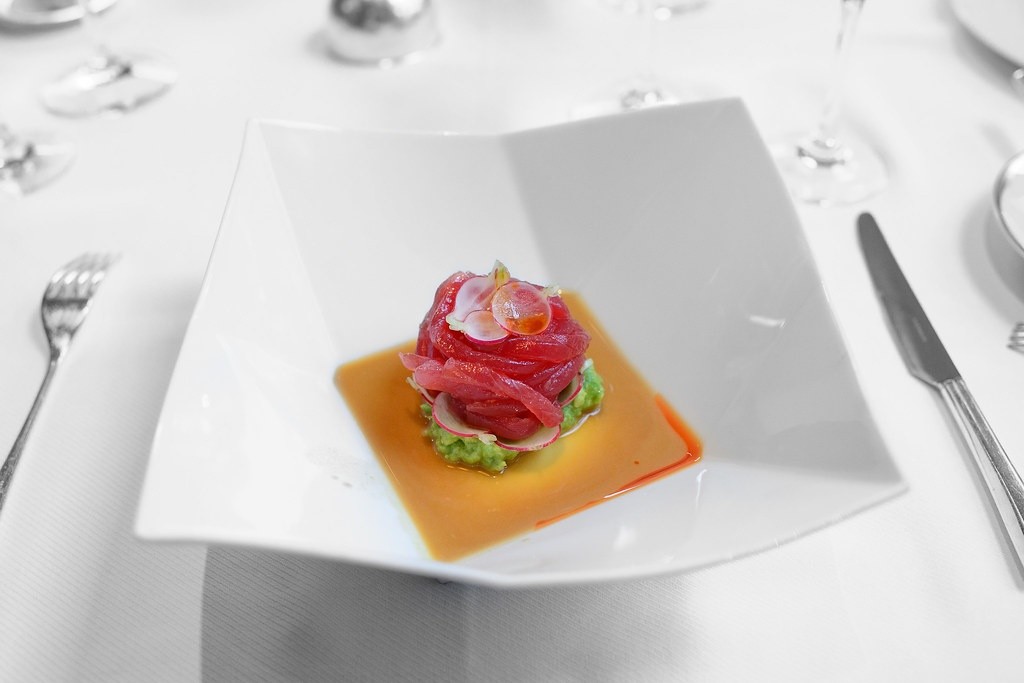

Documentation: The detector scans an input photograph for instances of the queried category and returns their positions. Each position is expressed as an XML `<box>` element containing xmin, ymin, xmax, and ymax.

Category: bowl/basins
<box><xmin>994</xmin><ymin>151</ymin><xmax>1024</xmax><ymax>259</ymax></box>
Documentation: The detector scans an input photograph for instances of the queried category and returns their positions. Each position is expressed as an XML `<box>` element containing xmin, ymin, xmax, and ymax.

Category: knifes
<box><xmin>853</xmin><ymin>214</ymin><xmax>1024</xmax><ymax>580</ymax></box>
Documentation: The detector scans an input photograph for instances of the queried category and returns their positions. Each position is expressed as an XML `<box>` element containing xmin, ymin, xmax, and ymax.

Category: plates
<box><xmin>134</xmin><ymin>97</ymin><xmax>910</xmax><ymax>591</ymax></box>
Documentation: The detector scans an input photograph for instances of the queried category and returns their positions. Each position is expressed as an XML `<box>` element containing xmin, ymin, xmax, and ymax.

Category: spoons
<box><xmin>1007</xmin><ymin>321</ymin><xmax>1024</xmax><ymax>347</ymax></box>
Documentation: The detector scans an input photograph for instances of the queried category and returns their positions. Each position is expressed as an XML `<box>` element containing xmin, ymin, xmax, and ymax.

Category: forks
<box><xmin>0</xmin><ymin>244</ymin><xmax>120</xmax><ymax>510</ymax></box>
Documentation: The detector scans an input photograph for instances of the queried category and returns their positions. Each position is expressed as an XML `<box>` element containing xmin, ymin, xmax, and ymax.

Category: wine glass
<box><xmin>44</xmin><ymin>0</ymin><xmax>169</xmax><ymax>113</ymax></box>
<box><xmin>770</xmin><ymin>0</ymin><xmax>901</xmax><ymax>211</ymax></box>
